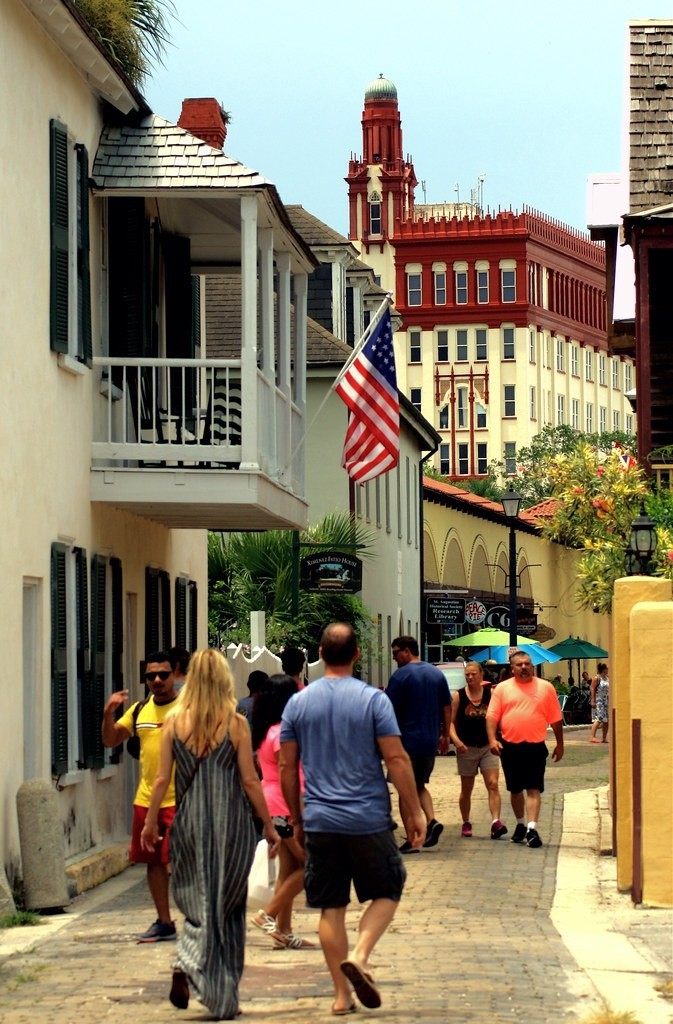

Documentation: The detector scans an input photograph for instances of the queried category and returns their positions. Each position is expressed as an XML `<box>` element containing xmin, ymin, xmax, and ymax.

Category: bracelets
<box><xmin>291</xmin><ymin>816</ymin><xmax>302</xmax><ymax>825</ymax></box>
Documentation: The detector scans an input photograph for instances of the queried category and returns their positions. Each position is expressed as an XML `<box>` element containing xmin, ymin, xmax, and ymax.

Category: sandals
<box><xmin>250</xmin><ymin>909</ymin><xmax>284</xmax><ymax>943</ymax></box>
<box><xmin>273</xmin><ymin>933</ymin><xmax>322</xmax><ymax>951</ymax></box>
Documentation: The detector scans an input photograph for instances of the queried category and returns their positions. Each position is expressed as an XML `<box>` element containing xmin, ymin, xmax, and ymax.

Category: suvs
<box><xmin>430</xmin><ymin>660</ymin><xmax>491</xmax><ymax>696</ymax></box>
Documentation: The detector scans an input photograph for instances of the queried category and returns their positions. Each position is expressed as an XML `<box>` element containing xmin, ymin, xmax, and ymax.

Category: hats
<box><xmin>245</xmin><ymin>839</ymin><xmax>280</xmax><ymax>904</ymax></box>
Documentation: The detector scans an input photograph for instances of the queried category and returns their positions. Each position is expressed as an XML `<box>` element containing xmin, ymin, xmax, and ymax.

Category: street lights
<box><xmin>500</xmin><ymin>482</ymin><xmax>523</xmax><ymax>648</ymax></box>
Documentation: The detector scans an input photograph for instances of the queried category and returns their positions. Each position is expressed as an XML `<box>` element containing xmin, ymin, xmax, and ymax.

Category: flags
<box><xmin>331</xmin><ymin>296</ymin><xmax>400</xmax><ymax>487</ymax></box>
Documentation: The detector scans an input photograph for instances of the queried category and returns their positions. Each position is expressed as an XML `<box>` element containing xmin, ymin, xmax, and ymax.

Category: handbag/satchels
<box><xmin>127</xmin><ymin>695</ymin><xmax>152</xmax><ymax>759</ymax></box>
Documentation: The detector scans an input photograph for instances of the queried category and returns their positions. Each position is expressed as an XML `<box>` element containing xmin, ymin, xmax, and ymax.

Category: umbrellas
<box><xmin>439</xmin><ymin>626</ymin><xmax>540</xmax><ymax>659</ymax></box>
<box><xmin>468</xmin><ymin>644</ymin><xmax>563</xmax><ymax>667</ymax></box>
<box><xmin>547</xmin><ymin>635</ymin><xmax>608</xmax><ymax>688</ymax></box>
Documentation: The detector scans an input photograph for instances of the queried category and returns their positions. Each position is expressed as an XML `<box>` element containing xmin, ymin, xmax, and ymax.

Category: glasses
<box><xmin>144</xmin><ymin>671</ymin><xmax>174</xmax><ymax>682</ymax></box>
<box><xmin>392</xmin><ymin>649</ymin><xmax>402</xmax><ymax>655</ymax></box>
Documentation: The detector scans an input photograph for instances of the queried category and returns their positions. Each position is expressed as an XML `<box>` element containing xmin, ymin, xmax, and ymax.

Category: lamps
<box><xmin>622</xmin><ymin>498</ymin><xmax>658</xmax><ymax>576</ymax></box>
<box><xmin>463</xmin><ymin>596</ymin><xmax>543</xmax><ymax>611</ymax></box>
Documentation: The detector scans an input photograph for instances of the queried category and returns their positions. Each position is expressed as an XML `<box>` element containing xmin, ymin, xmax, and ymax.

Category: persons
<box><xmin>554</xmin><ymin>672</ymin><xmax>593</xmax><ymax>704</ymax></box>
<box><xmin>101</xmin><ymin>644</ymin><xmax>316</xmax><ymax>1020</ymax></box>
<box><xmin>385</xmin><ymin>636</ymin><xmax>453</xmax><ymax>854</ymax></box>
<box><xmin>278</xmin><ymin>622</ymin><xmax>426</xmax><ymax>1015</ymax></box>
<box><xmin>450</xmin><ymin>662</ymin><xmax>508</xmax><ymax>840</ymax></box>
<box><xmin>485</xmin><ymin>651</ymin><xmax>564</xmax><ymax>848</ymax></box>
<box><xmin>588</xmin><ymin>662</ymin><xmax>609</xmax><ymax>743</ymax></box>
<box><xmin>456</xmin><ymin>656</ymin><xmax>464</xmax><ymax>666</ymax></box>
<box><xmin>484</xmin><ymin>659</ymin><xmax>510</xmax><ymax>683</ymax></box>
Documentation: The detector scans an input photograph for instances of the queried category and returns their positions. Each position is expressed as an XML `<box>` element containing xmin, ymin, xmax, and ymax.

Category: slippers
<box><xmin>340</xmin><ymin>959</ymin><xmax>382</xmax><ymax>1009</ymax></box>
<box><xmin>332</xmin><ymin>999</ymin><xmax>357</xmax><ymax>1015</ymax></box>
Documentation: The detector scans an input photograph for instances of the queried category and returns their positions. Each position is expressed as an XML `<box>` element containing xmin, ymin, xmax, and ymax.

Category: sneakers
<box><xmin>511</xmin><ymin>823</ymin><xmax>528</xmax><ymax>843</ymax></box>
<box><xmin>492</xmin><ymin>819</ymin><xmax>510</xmax><ymax>840</ymax></box>
<box><xmin>399</xmin><ymin>840</ymin><xmax>420</xmax><ymax>854</ymax></box>
<box><xmin>422</xmin><ymin>818</ymin><xmax>444</xmax><ymax>848</ymax></box>
<box><xmin>135</xmin><ymin>918</ymin><xmax>177</xmax><ymax>943</ymax></box>
<box><xmin>526</xmin><ymin>828</ymin><xmax>542</xmax><ymax>848</ymax></box>
<box><xmin>461</xmin><ymin>822</ymin><xmax>473</xmax><ymax>838</ymax></box>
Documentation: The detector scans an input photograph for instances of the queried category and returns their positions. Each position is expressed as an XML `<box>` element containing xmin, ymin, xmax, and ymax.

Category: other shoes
<box><xmin>169</xmin><ymin>966</ymin><xmax>190</xmax><ymax>1009</ymax></box>
<box><xmin>590</xmin><ymin>738</ymin><xmax>609</xmax><ymax>743</ymax></box>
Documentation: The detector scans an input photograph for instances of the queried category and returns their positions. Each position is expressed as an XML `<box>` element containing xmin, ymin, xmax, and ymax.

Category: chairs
<box><xmin>125</xmin><ymin>365</ymin><xmax>210</xmax><ymax>468</ymax></box>
<box><xmin>549</xmin><ymin>690</ymin><xmax>591</xmax><ymax>727</ymax></box>
